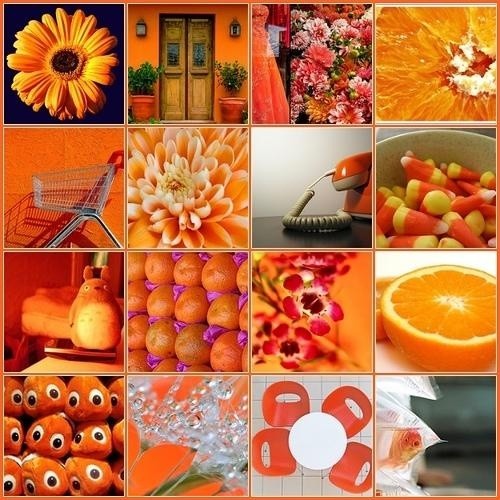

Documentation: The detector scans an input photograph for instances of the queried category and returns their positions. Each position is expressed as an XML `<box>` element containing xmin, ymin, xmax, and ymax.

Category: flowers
<box><xmin>291</xmin><ymin>4</ymin><xmax>372</xmax><ymax>125</ymax></box>
<box><xmin>6</xmin><ymin>7</ymin><xmax>120</xmax><ymax>122</ymax></box>
<box><xmin>253</xmin><ymin>251</ymin><xmax>362</xmax><ymax>373</ymax></box>
<box><xmin>126</xmin><ymin>128</ymin><xmax>249</xmax><ymax>249</ymax></box>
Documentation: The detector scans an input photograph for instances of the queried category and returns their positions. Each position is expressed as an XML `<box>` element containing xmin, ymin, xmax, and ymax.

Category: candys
<box><xmin>376</xmin><ymin>150</ymin><xmax>496</xmax><ymax>248</ymax></box>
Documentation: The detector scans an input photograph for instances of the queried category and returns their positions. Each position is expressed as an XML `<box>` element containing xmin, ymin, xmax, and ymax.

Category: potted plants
<box><xmin>128</xmin><ymin>60</ymin><xmax>166</xmax><ymax>121</ymax></box>
<box><xmin>213</xmin><ymin>59</ymin><xmax>248</xmax><ymax>124</ymax></box>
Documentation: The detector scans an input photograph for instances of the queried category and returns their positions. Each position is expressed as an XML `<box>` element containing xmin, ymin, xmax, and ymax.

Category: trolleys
<box><xmin>32</xmin><ymin>150</ymin><xmax>123</xmax><ymax>248</ymax></box>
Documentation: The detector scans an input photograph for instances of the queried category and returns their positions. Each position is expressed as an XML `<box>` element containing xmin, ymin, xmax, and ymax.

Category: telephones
<box><xmin>331</xmin><ymin>151</ymin><xmax>373</xmax><ymax>217</ymax></box>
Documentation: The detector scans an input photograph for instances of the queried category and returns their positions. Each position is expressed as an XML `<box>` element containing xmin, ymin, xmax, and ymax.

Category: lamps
<box><xmin>135</xmin><ymin>18</ymin><xmax>146</xmax><ymax>36</ymax></box>
<box><xmin>229</xmin><ymin>16</ymin><xmax>241</xmax><ymax>36</ymax></box>
